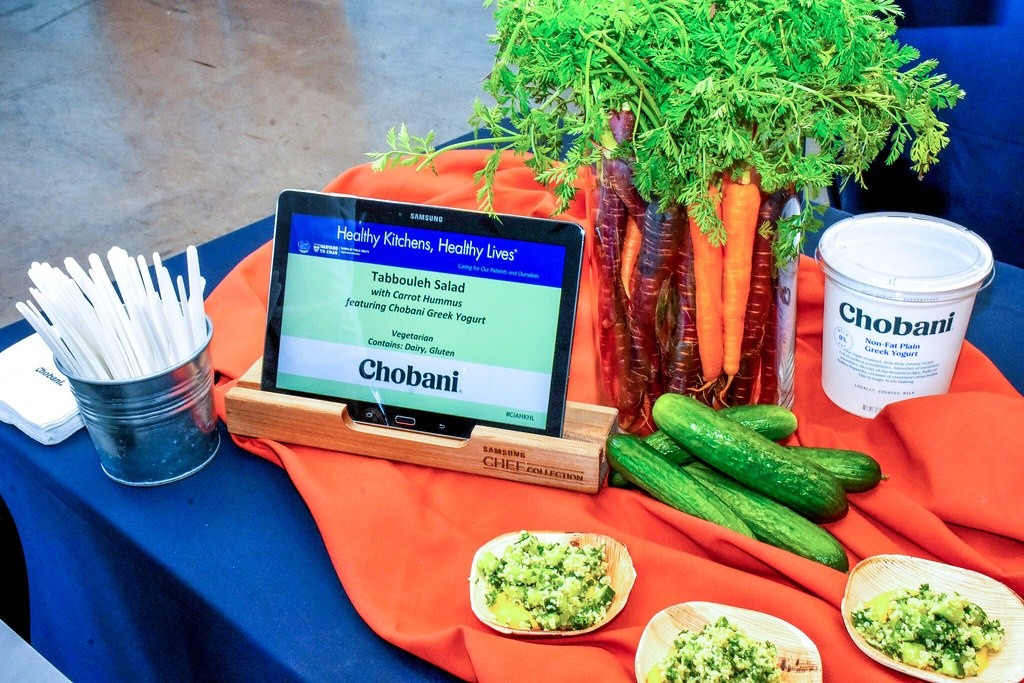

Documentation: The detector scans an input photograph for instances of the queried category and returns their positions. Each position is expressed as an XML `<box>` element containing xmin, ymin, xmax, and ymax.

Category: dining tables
<box><xmin>0</xmin><ymin>108</ymin><xmax>1023</xmax><ymax>683</ymax></box>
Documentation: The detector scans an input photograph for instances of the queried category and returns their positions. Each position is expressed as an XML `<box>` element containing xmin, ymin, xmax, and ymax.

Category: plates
<box><xmin>842</xmin><ymin>554</ymin><xmax>1022</xmax><ymax>683</ymax></box>
<box><xmin>469</xmin><ymin>527</ymin><xmax>636</xmax><ymax>637</ymax></box>
<box><xmin>632</xmin><ymin>602</ymin><xmax>823</xmax><ymax>683</ymax></box>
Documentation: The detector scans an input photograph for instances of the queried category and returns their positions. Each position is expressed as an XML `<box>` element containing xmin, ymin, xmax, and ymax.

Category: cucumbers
<box><xmin>605</xmin><ymin>393</ymin><xmax>890</xmax><ymax>574</ymax></box>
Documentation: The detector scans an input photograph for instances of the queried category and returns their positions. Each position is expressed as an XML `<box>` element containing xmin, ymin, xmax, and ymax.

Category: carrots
<box><xmin>362</xmin><ymin>48</ymin><xmax>972</xmax><ymax>434</ymax></box>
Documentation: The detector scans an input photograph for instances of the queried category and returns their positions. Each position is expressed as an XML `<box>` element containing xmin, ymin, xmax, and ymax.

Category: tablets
<box><xmin>259</xmin><ymin>188</ymin><xmax>586</xmax><ymax>443</ymax></box>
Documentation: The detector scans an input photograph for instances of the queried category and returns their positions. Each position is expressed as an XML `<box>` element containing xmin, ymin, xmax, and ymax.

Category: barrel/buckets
<box><xmin>813</xmin><ymin>210</ymin><xmax>993</xmax><ymax>420</ymax></box>
<box><xmin>53</xmin><ymin>314</ymin><xmax>220</xmax><ymax>488</ymax></box>
